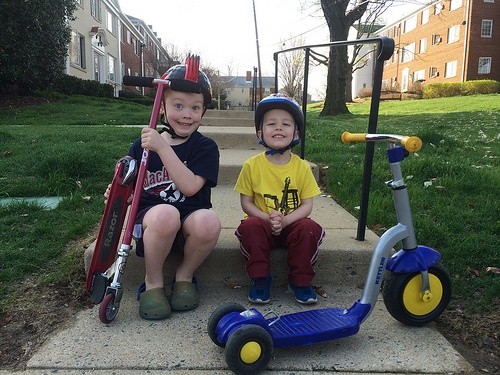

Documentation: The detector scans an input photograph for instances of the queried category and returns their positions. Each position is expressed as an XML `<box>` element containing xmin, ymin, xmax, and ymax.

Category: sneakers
<box><xmin>287</xmin><ymin>280</ymin><xmax>318</xmax><ymax>304</ymax></box>
<box><xmin>248</xmin><ymin>276</ymin><xmax>273</xmax><ymax>304</ymax></box>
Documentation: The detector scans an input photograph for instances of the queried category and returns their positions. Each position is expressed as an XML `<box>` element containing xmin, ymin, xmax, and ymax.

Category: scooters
<box><xmin>207</xmin><ymin>132</ymin><xmax>453</xmax><ymax>375</ymax></box>
<box><xmin>83</xmin><ymin>75</ymin><xmax>203</xmax><ymax>324</ymax></box>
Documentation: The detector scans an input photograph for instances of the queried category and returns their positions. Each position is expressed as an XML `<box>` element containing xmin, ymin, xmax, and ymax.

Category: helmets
<box><xmin>255</xmin><ymin>93</ymin><xmax>306</xmax><ymax>155</ymax></box>
<box><xmin>158</xmin><ymin>53</ymin><xmax>215</xmax><ymax>109</ymax></box>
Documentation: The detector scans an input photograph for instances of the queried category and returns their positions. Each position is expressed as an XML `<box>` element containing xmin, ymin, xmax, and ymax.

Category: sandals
<box><xmin>135</xmin><ymin>282</ymin><xmax>172</xmax><ymax>321</ymax></box>
<box><xmin>170</xmin><ymin>270</ymin><xmax>199</xmax><ymax>312</ymax></box>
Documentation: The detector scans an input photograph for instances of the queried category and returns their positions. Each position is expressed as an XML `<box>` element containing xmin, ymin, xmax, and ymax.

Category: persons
<box><xmin>234</xmin><ymin>93</ymin><xmax>326</xmax><ymax>305</ymax></box>
<box><xmin>104</xmin><ymin>65</ymin><xmax>221</xmax><ymax>320</ymax></box>
